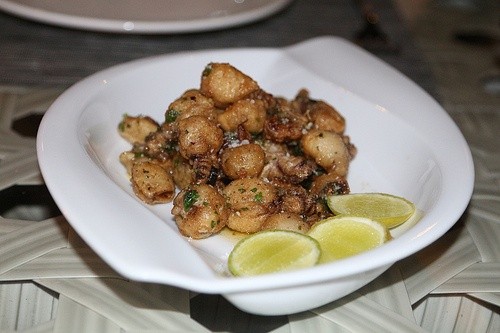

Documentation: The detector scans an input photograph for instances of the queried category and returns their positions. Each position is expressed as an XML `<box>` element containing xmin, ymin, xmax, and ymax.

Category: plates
<box><xmin>36</xmin><ymin>36</ymin><xmax>474</xmax><ymax>315</ymax></box>
<box><xmin>0</xmin><ymin>0</ymin><xmax>292</xmax><ymax>35</ymax></box>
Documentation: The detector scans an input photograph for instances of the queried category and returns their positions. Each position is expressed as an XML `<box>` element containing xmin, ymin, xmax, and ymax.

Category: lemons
<box><xmin>306</xmin><ymin>214</ymin><xmax>392</xmax><ymax>265</ymax></box>
<box><xmin>228</xmin><ymin>229</ymin><xmax>321</xmax><ymax>277</ymax></box>
<box><xmin>325</xmin><ymin>193</ymin><xmax>416</xmax><ymax>229</ymax></box>
